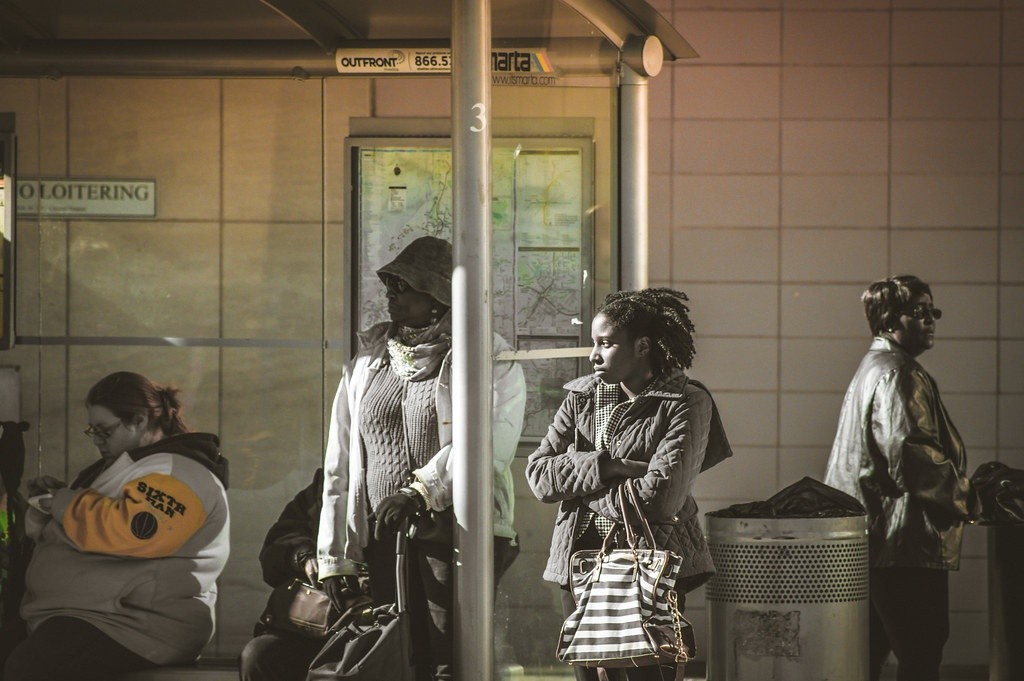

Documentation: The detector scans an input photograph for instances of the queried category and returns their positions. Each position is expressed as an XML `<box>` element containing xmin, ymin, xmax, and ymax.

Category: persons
<box><xmin>0</xmin><ymin>371</ymin><xmax>230</xmax><ymax>681</ymax></box>
<box><xmin>311</xmin><ymin>236</ymin><xmax>527</xmax><ymax>681</ymax></box>
<box><xmin>239</xmin><ymin>467</ymin><xmax>325</xmax><ymax>681</ymax></box>
<box><xmin>525</xmin><ymin>285</ymin><xmax>733</xmax><ymax>681</ymax></box>
<box><xmin>823</xmin><ymin>275</ymin><xmax>1000</xmax><ymax>681</ymax></box>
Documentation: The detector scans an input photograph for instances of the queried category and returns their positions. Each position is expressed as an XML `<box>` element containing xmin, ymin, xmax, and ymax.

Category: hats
<box><xmin>376</xmin><ymin>236</ymin><xmax>452</xmax><ymax>307</ymax></box>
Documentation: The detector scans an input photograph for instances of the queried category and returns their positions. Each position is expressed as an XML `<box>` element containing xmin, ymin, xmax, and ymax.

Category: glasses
<box><xmin>385</xmin><ymin>277</ymin><xmax>410</xmax><ymax>294</ymax></box>
<box><xmin>898</xmin><ymin>307</ymin><xmax>942</xmax><ymax>320</ymax></box>
<box><xmin>85</xmin><ymin>413</ymin><xmax>136</xmax><ymax>439</ymax></box>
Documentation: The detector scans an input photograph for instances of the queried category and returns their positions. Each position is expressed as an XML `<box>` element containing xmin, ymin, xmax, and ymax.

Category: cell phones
<box><xmin>27</xmin><ymin>493</ymin><xmax>53</xmax><ymax>515</ymax></box>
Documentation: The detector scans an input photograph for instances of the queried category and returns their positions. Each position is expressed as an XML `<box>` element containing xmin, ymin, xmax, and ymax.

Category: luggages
<box><xmin>306</xmin><ymin>515</ymin><xmax>413</xmax><ymax>681</ymax></box>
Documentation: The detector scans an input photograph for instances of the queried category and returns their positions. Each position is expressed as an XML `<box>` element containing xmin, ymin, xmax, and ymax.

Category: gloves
<box><xmin>19</xmin><ymin>475</ymin><xmax>68</xmax><ymax>514</ymax></box>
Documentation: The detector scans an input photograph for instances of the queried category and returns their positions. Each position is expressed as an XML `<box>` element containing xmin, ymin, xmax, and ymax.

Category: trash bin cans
<box><xmin>704</xmin><ymin>511</ymin><xmax>881</xmax><ymax>681</ymax></box>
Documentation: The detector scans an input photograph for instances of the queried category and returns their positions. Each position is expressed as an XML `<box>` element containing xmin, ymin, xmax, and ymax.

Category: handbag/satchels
<box><xmin>969</xmin><ymin>461</ymin><xmax>1024</xmax><ymax>527</ymax></box>
<box><xmin>261</xmin><ymin>574</ymin><xmax>336</xmax><ymax>641</ymax></box>
<box><xmin>557</xmin><ymin>478</ymin><xmax>697</xmax><ymax>668</ymax></box>
